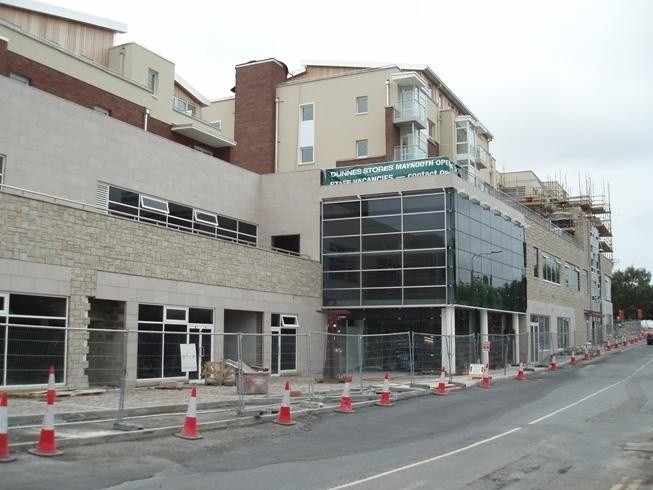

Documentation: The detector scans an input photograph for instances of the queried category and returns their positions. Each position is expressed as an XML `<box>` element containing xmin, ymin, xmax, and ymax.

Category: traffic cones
<box><xmin>548</xmin><ymin>331</ymin><xmax>648</xmax><ymax>370</ymax></box>
<box><xmin>26</xmin><ymin>388</ymin><xmax>65</xmax><ymax>456</ymax></box>
<box><xmin>515</xmin><ymin>360</ymin><xmax>526</xmax><ymax>380</ymax></box>
<box><xmin>431</xmin><ymin>366</ymin><xmax>449</xmax><ymax>396</ymax></box>
<box><xmin>0</xmin><ymin>390</ymin><xmax>18</xmax><ymax>464</ymax></box>
<box><xmin>172</xmin><ymin>386</ymin><xmax>203</xmax><ymax>440</ymax></box>
<box><xmin>334</xmin><ymin>375</ymin><xmax>355</xmax><ymax>414</ymax></box>
<box><xmin>41</xmin><ymin>365</ymin><xmax>62</xmax><ymax>402</ymax></box>
<box><xmin>374</xmin><ymin>371</ymin><xmax>394</xmax><ymax>407</ymax></box>
<box><xmin>271</xmin><ymin>380</ymin><xmax>296</xmax><ymax>426</ymax></box>
<box><xmin>478</xmin><ymin>363</ymin><xmax>491</xmax><ymax>388</ymax></box>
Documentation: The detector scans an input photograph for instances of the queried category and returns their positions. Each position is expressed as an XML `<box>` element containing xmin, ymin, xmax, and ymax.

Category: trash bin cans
<box><xmin>235</xmin><ymin>366</ymin><xmax>270</xmax><ymax>395</ymax></box>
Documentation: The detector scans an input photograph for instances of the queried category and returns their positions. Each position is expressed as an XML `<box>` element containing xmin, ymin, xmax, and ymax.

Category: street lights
<box><xmin>469</xmin><ymin>250</ymin><xmax>504</xmax><ymax>289</ymax></box>
